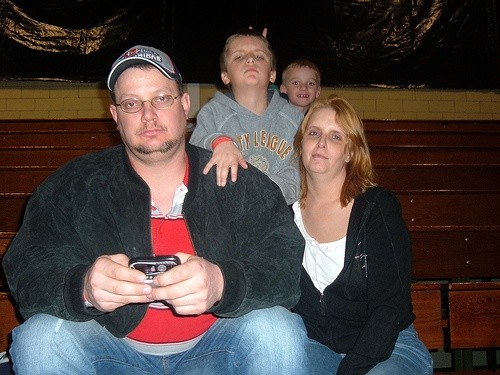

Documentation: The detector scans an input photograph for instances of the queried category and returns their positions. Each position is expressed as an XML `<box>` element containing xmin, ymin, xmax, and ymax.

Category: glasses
<box><xmin>114</xmin><ymin>94</ymin><xmax>181</xmax><ymax>113</ymax></box>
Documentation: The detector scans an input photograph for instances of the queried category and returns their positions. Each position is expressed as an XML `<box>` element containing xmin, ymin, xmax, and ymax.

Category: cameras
<box><xmin>129</xmin><ymin>254</ymin><xmax>181</xmax><ymax>282</ymax></box>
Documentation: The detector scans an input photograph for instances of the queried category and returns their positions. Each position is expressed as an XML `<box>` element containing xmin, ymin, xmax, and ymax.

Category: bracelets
<box><xmin>210</xmin><ymin>135</ymin><xmax>233</xmax><ymax>151</ymax></box>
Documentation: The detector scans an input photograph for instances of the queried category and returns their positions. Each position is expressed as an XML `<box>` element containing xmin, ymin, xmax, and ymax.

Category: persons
<box><xmin>287</xmin><ymin>95</ymin><xmax>434</xmax><ymax>375</ymax></box>
<box><xmin>188</xmin><ymin>29</ymin><xmax>305</xmax><ymax>222</ymax></box>
<box><xmin>248</xmin><ymin>27</ymin><xmax>322</xmax><ymax>116</ymax></box>
<box><xmin>1</xmin><ymin>44</ymin><xmax>308</xmax><ymax>375</ymax></box>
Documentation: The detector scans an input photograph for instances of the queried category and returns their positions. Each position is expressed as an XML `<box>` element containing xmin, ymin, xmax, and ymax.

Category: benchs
<box><xmin>0</xmin><ymin>119</ymin><xmax>500</xmax><ymax>375</ymax></box>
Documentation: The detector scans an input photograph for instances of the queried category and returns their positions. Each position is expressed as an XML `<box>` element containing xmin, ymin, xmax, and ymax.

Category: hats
<box><xmin>106</xmin><ymin>45</ymin><xmax>182</xmax><ymax>92</ymax></box>
